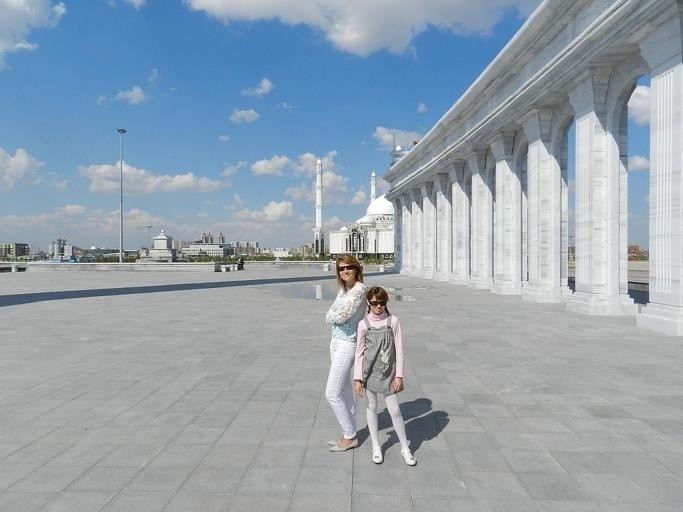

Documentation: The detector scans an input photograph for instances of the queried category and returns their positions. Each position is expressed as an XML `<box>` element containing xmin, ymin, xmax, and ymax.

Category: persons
<box><xmin>322</xmin><ymin>254</ymin><xmax>370</xmax><ymax>454</ymax></box>
<box><xmin>350</xmin><ymin>284</ymin><xmax>418</xmax><ymax>468</ymax></box>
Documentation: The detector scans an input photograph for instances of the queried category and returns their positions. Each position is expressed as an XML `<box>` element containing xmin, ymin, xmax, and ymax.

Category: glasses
<box><xmin>369</xmin><ymin>300</ymin><xmax>387</xmax><ymax>306</ymax></box>
<box><xmin>337</xmin><ymin>265</ymin><xmax>356</xmax><ymax>271</ymax></box>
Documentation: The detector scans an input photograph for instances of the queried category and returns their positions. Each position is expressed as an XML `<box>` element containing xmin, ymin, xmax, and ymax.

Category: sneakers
<box><xmin>401</xmin><ymin>449</ymin><xmax>416</xmax><ymax>466</ymax></box>
<box><xmin>371</xmin><ymin>447</ymin><xmax>383</xmax><ymax>464</ymax></box>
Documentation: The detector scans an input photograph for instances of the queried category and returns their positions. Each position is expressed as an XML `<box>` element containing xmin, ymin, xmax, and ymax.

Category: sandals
<box><xmin>328</xmin><ymin>439</ymin><xmax>359</xmax><ymax>452</ymax></box>
